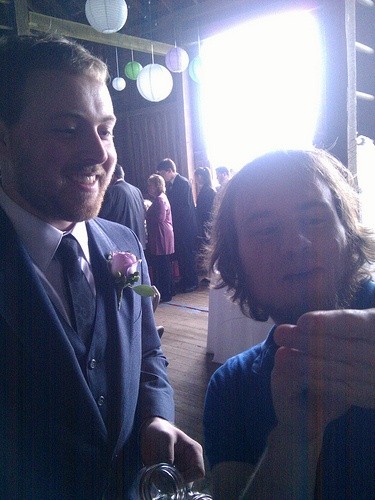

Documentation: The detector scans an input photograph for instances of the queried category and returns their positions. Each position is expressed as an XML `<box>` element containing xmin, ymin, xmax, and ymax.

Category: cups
<box><xmin>129</xmin><ymin>463</ymin><xmax>187</xmax><ymax>500</ymax></box>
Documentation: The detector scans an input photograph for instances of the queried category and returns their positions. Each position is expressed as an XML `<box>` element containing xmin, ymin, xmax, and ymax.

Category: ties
<box><xmin>54</xmin><ymin>233</ymin><xmax>97</xmax><ymax>347</ymax></box>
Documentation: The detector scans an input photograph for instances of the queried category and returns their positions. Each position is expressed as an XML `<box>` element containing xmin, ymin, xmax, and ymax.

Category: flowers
<box><xmin>107</xmin><ymin>251</ymin><xmax>155</xmax><ymax>310</ymax></box>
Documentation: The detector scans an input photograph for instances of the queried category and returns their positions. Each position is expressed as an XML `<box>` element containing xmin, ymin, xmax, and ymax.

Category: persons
<box><xmin>203</xmin><ymin>149</ymin><xmax>375</xmax><ymax>500</ymax></box>
<box><xmin>102</xmin><ymin>158</ymin><xmax>233</xmax><ymax>300</ymax></box>
<box><xmin>0</xmin><ymin>32</ymin><xmax>205</xmax><ymax>500</ymax></box>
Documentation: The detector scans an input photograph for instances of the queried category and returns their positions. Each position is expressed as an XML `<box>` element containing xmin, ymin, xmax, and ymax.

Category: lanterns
<box><xmin>113</xmin><ymin>48</ymin><xmax>210</xmax><ymax>102</ymax></box>
<box><xmin>85</xmin><ymin>0</ymin><xmax>128</xmax><ymax>33</ymax></box>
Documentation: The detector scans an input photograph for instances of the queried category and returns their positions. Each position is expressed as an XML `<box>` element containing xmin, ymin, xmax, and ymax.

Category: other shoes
<box><xmin>177</xmin><ymin>279</ymin><xmax>197</xmax><ymax>293</ymax></box>
